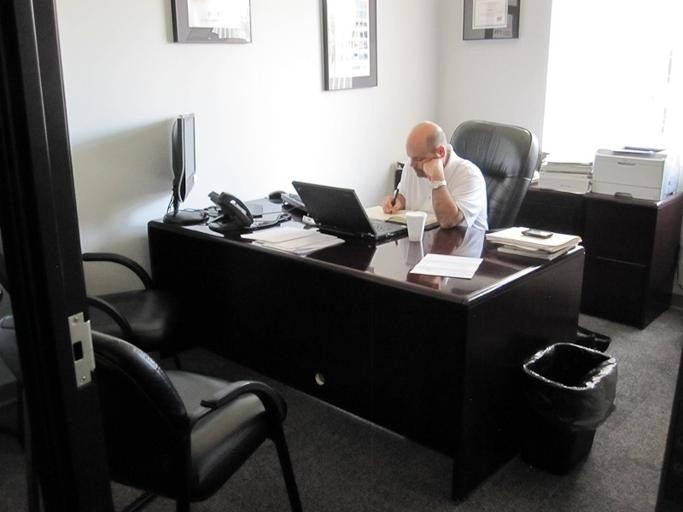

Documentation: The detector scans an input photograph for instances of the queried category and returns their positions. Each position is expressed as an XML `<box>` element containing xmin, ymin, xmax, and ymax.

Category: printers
<box><xmin>591</xmin><ymin>148</ymin><xmax>679</xmax><ymax>201</ymax></box>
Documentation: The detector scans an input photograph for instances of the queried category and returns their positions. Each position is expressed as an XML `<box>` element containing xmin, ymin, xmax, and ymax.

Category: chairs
<box><xmin>87</xmin><ymin>329</ymin><xmax>304</xmax><ymax>511</ymax></box>
<box><xmin>448</xmin><ymin>119</ymin><xmax>542</xmax><ymax>233</ymax></box>
<box><xmin>0</xmin><ymin>283</ymin><xmax>27</xmax><ymax>453</ymax></box>
<box><xmin>81</xmin><ymin>249</ymin><xmax>182</xmax><ymax>373</ymax></box>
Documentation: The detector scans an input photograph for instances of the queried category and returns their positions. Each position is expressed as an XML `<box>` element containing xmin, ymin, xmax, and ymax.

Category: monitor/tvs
<box><xmin>164</xmin><ymin>113</ymin><xmax>209</xmax><ymax>225</ymax></box>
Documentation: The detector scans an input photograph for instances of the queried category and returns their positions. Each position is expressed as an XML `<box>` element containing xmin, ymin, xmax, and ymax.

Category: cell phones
<box><xmin>521</xmin><ymin>228</ymin><xmax>553</xmax><ymax>238</ymax></box>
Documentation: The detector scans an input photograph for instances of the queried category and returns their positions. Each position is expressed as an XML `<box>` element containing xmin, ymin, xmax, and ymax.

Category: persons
<box><xmin>382</xmin><ymin>121</ymin><xmax>489</xmax><ymax>230</ymax></box>
<box><xmin>400</xmin><ymin>227</ymin><xmax>485</xmax><ymax>290</ymax></box>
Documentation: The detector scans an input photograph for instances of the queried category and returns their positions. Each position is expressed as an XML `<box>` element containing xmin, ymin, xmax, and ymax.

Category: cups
<box><xmin>407</xmin><ymin>242</ymin><xmax>426</xmax><ymax>268</ymax></box>
<box><xmin>405</xmin><ymin>209</ymin><xmax>428</xmax><ymax>242</ymax></box>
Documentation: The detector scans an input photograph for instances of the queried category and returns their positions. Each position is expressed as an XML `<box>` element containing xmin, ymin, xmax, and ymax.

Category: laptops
<box><xmin>292</xmin><ymin>181</ymin><xmax>407</xmax><ymax>241</ymax></box>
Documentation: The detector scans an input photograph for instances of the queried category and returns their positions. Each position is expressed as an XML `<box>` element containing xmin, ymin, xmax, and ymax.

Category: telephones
<box><xmin>208</xmin><ymin>190</ymin><xmax>254</xmax><ymax>227</ymax></box>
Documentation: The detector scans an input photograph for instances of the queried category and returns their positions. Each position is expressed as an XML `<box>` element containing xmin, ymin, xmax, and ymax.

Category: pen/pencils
<box><xmin>391</xmin><ymin>188</ymin><xmax>399</xmax><ymax>214</ymax></box>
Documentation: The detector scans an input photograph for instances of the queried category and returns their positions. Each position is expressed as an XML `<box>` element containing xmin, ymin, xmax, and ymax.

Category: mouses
<box><xmin>269</xmin><ymin>190</ymin><xmax>287</xmax><ymax>203</ymax></box>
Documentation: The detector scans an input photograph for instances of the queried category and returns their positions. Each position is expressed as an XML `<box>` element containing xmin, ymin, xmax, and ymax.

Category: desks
<box><xmin>147</xmin><ymin>197</ymin><xmax>594</xmax><ymax>504</ymax></box>
<box><xmin>393</xmin><ymin>162</ymin><xmax>683</xmax><ymax>333</ymax></box>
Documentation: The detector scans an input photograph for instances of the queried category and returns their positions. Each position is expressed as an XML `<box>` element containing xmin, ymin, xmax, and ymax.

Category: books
<box><xmin>368</xmin><ymin>206</ymin><xmax>440</xmax><ymax>231</ymax></box>
<box><xmin>485</xmin><ymin>226</ymin><xmax>582</xmax><ymax>262</ymax></box>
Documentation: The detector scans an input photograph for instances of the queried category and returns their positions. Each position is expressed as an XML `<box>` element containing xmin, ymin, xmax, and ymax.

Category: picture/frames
<box><xmin>461</xmin><ymin>0</ymin><xmax>521</xmax><ymax>41</ymax></box>
<box><xmin>320</xmin><ymin>0</ymin><xmax>378</xmax><ymax>91</ymax></box>
<box><xmin>169</xmin><ymin>0</ymin><xmax>252</xmax><ymax>46</ymax></box>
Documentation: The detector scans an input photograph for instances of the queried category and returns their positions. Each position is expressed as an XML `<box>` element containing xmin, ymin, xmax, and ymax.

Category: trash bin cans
<box><xmin>512</xmin><ymin>340</ymin><xmax>617</xmax><ymax>474</ymax></box>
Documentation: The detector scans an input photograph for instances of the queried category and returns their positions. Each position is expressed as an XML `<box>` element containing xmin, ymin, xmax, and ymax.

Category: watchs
<box><xmin>431</xmin><ymin>180</ymin><xmax>447</xmax><ymax>189</ymax></box>
